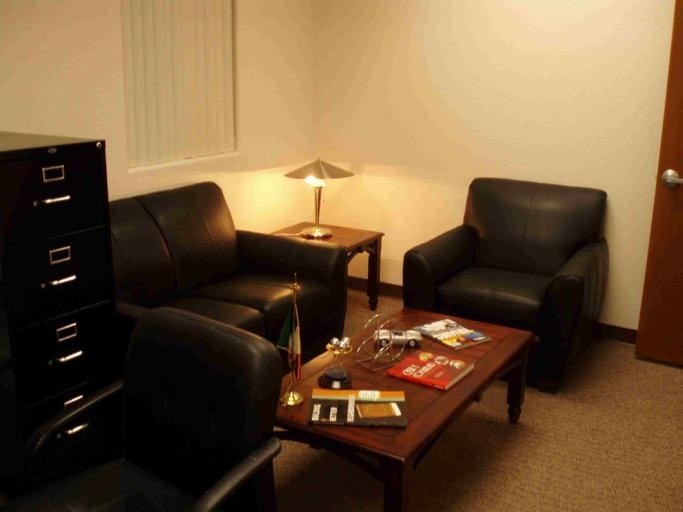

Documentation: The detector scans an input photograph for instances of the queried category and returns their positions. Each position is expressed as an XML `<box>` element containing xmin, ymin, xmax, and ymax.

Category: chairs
<box><xmin>402</xmin><ymin>177</ymin><xmax>610</xmax><ymax>394</ymax></box>
<box><xmin>26</xmin><ymin>307</ymin><xmax>284</xmax><ymax>510</ymax></box>
<box><xmin>109</xmin><ymin>181</ymin><xmax>351</xmax><ymax>375</ymax></box>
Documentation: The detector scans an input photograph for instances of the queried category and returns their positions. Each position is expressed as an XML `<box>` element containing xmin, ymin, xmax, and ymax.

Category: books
<box><xmin>386</xmin><ymin>348</ymin><xmax>474</xmax><ymax>392</ymax></box>
<box><xmin>414</xmin><ymin>319</ymin><xmax>492</xmax><ymax>350</ymax></box>
<box><xmin>307</xmin><ymin>387</ymin><xmax>408</xmax><ymax>427</ymax></box>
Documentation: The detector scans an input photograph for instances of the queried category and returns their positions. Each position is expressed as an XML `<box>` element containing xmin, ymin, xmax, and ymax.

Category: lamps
<box><xmin>284</xmin><ymin>156</ymin><xmax>356</xmax><ymax>238</ymax></box>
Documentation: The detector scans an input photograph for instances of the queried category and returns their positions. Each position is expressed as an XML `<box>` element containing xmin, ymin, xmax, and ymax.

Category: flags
<box><xmin>276</xmin><ymin>296</ymin><xmax>301</xmax><ymax>384</ymax></box>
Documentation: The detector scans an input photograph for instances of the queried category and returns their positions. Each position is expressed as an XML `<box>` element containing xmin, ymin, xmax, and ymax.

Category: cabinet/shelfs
<box><xmin>0</xmin><ymin>140</ymin><xmax>120</xmax><ymax>500</ymax></box>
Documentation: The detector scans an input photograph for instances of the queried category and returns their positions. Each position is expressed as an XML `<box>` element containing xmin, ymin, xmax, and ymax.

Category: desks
<box><xmin>269</xmin><ymin>222</ymin><xmax>385</xmax><ymax>309</ymax></box>
